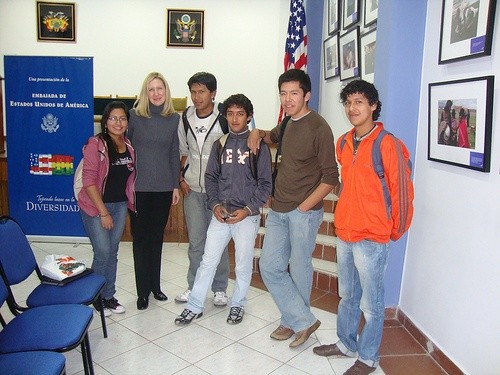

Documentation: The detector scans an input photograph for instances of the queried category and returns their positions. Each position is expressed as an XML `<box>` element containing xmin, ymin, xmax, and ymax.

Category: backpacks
<box><xmin>74</xmin><ymin>137</ymin><xmax>131</xmax><ymax>201</ymax></box>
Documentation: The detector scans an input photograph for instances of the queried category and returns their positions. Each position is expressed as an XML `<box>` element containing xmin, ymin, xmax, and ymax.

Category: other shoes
<box><xmin>137</xmin><ymin>296</ymin><xmax>148</xmax><ymax>310</ymax></box>
<box><xmin>343</xmin><ymin>359</ymin><xmax>376</xmax><ymax>375</ymax></box>
<box><xmin>290</xmin><ymin>319</ymin><xmax>321</xmax><ymax>348</ymax></box>
<box><xmin>270</xmin><ymin>325</ymin><xmax>295</xmax><ymax>340</ymax></box>
<box><xmin>152</xmin><ymin>288</ymin><xmax>168</xmax><ymax>301</ymax></box>
<box><xmin>313</xmin><ymin>343</ymin><xmax>347</xmax><ymax>357</ymax></box>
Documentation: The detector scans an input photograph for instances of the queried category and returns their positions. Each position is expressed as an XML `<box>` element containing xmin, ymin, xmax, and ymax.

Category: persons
<box><xmin>246</xmin><ymin>69</ymin><xmax>338</xmax><ymax>349</ymax></box>
<box><xmin>123</xmin><ymin>71</ymin><xmax>186</xmax><ymax>310</ymax></box>
<box><xmin>79</xmin><ymin>100</ymin><xmax>135</xmax><ymax>319</ymax></box>
<box><xmin>173</xmin><ymin>94</ymin><xmax>271</xmax><ymax>325</ymax></box>
<box><xmin>312</xmin><ymin>80</ymin><xmax>415</xmax><ymax>375</ymax></box>
<box><xmin>438</xmin><ymin>101</ymin><xmax>472</xmax><ymax>148</ymax></box>
<box><xmin>175</xmin><ymin>72</ymin><xmax>231</xmax><ymax>310</ymax></box>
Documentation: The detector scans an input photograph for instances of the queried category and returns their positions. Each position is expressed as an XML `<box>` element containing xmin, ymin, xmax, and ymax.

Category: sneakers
<box><xmin>175</xmin><ymin>309</ymin><xmax>203</xmax><ymax>325</ymax></box>
<box><xmin>104</xmin><ymin>297</ymin><xmax>125</xmax><ymax>313</ymax></box>
<box><xmin>174</xmin><ymin>289</ymin><xmax>191</xmax><ymax>302</ymax></box>
<box><xmin>214</xmin><ymin>291</ymin><xmax>227</xmax><ymax>306</ymax></box>
<box><xmin>91</xmin><ymin>295</ymin><xmax>111</xmax><ymax>316</ymax></box>
<box><xmin>227</xmin><ymin>306</ymin><xmax>244</xmax><ymax>324</ymax></box>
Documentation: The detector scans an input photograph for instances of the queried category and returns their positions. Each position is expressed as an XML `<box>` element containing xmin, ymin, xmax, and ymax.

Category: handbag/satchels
<box><xmin>270</xmin><ymin>116</ymin><xmax>291</xmax><ymax>197</ymax></box>
<box><xmin>41</xmin><ymin>267</ymin><xmax>95</xmax><ymax>287</ymax></box>
<box><xmin>39</xmin><ymin>252</ymin><xmax>86</xmax><ymax>281</ymax></box>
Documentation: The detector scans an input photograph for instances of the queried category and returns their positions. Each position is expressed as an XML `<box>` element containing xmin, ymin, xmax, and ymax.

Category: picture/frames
<box><xmin>323</xmin><ymin>0</ymin><xmax>378</xmax><ymax>85</ymax></box>
<box><xmin>167</xmin><ymin>9</ymin><xmax>204</xmax><ymax>48</ymax></box>
<box><xmin>438</xmin><ymin>0</ymin><xmax>497</xmax><ymax>65</ymax></box>
<box><xmin>37</xmin><ymin>1</ymin><xmax>75</xmax><ymax>41</ymax></box>
<box><xmin>427</xmin><ymin>76</ymin><xmax>494</xmax><ymax>172</ymax></box>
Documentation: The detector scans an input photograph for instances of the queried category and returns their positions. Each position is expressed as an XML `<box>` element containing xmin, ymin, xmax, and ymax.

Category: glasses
<box><xmin>108</xmin><ymin>115</ymin><xmax>127</xmax><ymax>122</ymax></box>
<box><xmin>342</xmin><ymin>97</ymin><xmax>366</xmax><ymax>107</ymax></box>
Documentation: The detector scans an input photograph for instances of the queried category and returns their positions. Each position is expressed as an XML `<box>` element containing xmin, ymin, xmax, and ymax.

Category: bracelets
<box><xmin>179</xmin><ymin>179</ymin><xmax>185</xmax><ymax>184</ymax></box>
<box><xmin>102</xmin><ymin>213</ymin><xmax>109</xmax><ymax>217</ymax></box>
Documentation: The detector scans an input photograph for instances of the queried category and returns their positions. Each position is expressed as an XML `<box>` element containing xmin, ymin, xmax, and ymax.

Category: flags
<box><xmin>278</xmin><ymin>0</ymin><xmax>307</xmax><ymax>126</ymax></box>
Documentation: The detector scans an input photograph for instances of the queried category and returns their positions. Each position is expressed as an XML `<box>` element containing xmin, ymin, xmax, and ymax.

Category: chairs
<box><xmin>0</xmin><ymin>216</ymin><xmax>108</xmax><ymax>375</ymax></box>
